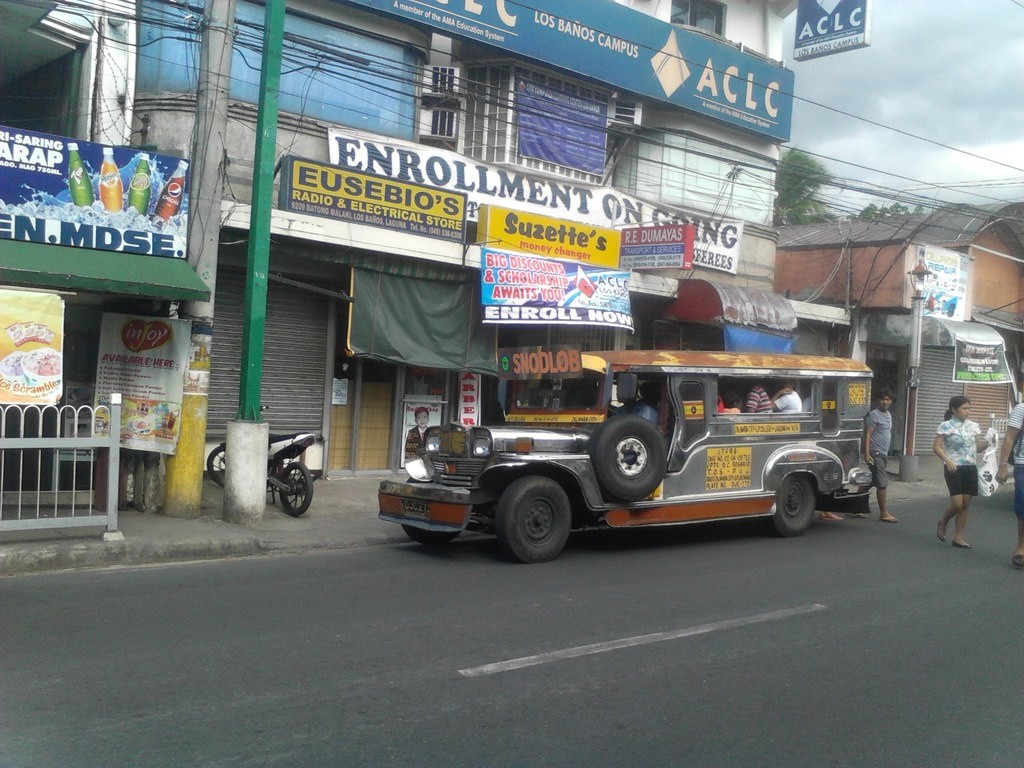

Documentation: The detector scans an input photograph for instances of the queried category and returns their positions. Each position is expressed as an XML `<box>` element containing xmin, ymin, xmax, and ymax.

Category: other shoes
<box><xmin>880</xmin><ymin>515</ymin><xmax>898</xmax><ymax>523</ymax></box>
<box><xmin>1011</xmin><ymin>550</ymin><xmax>1024</xmax><ymax>569</ymax></box>
<box><xmin>952</xmin><ymin>539</ymin><xmax>972</xmax><ymax>548</ymax></box>
<box><xmin>937</xmin><ymin>519</ymin><xmax>947</xmax><ymax>541</ymax></box>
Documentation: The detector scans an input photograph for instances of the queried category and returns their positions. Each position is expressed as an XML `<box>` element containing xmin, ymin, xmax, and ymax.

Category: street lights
<box><xmin>899</xmin><ymin>257</ymin><xmax>932</xmax><ymax>481</ymax></box>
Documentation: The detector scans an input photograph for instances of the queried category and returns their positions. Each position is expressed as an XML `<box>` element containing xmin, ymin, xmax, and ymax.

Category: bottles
<box><xmin>155</xmin><ymin>160</ymin><xmax>188</xmax><ymax>230</ymax></box>
<box><xmin>128</xmin><ymin>152</ymin><xmax>151</xmax><ymax>216</ymax></box>
<box><xmin>68</xmin><ymin>143</ymin><xmax>93</xmax><ymax>207</ymax></box>
<box><xmin>99</xmin><ymin>147</ymin><xmax>123</xmax><ymax>213</ymax></box>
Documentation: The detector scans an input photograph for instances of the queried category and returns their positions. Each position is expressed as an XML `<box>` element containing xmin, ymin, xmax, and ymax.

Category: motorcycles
<box><xmin>206</xmin><ymin>404</ymin><xmax>317</xmax><ymax>517</ymax></box>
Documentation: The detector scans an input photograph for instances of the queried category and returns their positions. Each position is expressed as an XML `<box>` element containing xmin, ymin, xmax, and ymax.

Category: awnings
<box><xmin>922</xmin><ymin>315</ymin><xmax>1007</xmax><ymax>351</ymax></box>
<box><xmin>0</xmin><ymin>238</ymin><xmax>210</xmax><ymax>301</ymax></box>
<box><xmin>664</xmin><ymin>278</ymin><xmax>798</xmax><ymax>332</ymax></box>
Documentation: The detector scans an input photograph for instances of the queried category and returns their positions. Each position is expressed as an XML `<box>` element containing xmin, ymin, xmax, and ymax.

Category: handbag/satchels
<box><xmin>976</xmin><ymin>434</ymin><xmax>999</xmax><ymax>496</ymax></box>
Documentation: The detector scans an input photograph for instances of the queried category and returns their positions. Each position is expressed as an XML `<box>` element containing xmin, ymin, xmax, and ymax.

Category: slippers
<box><xmin>819</xmin><ymin>514</ymin><xmax>843</xmax><ymax>521</ymax></box>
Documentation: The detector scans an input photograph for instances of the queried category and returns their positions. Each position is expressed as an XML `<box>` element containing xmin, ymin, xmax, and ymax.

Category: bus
<box><xmin>378</xmin><ymin>348</ymin><xmax>877</xmax><ymax>570</ymax></box>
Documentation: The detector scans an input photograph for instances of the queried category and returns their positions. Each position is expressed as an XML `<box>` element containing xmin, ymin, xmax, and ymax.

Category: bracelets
<box><xmin>999</xmin><ymin>463</ymin><xmax>1007</xmax><ymax>466</ymax></box>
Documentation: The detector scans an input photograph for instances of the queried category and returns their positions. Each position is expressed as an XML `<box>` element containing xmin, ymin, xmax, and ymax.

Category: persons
<box><xmin>770</xmin><ymin>379</ymin><xmax>802</xmax><ymax>413</ymax></box>
<box><xmin>996</xmin><ymin>402</ymin><xmax>1024</xmax><ymax>570</ymax></box>
<box><xmin>405</xmin><ymin>407</ymin><xmax>429</xmax><ymax>459</ymax></box>
<box><xmin>745</xmin><ymin>379</ymin><xmax>773</xmax><ymax>413</ymax></box>
<box><xmin>820</xmin><ymin>512</ymin><xmax>843</xmax><ymax>521</ymax></box>
<box><xmin>934</xmin><ymin>395</ymin><xmax>998</xmax><ymax>548</ymax></box>
<box><xmin>614</xmin><ymin>380</ymin><xmax>659</xmax><ymax>426</ymax></box>
<box><xmin>846</xmin><ymin>388</ymin><xmax>899</xmax><ymax>522</ymax></box>
